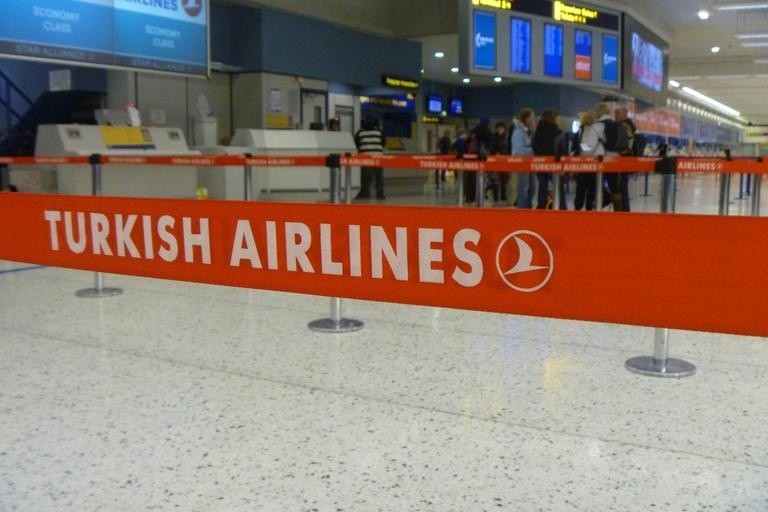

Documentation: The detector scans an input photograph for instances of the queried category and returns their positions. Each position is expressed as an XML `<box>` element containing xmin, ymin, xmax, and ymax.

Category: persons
<box><xmin>632</xmin><ymin>40</ymin><xmax>648</xmax><ymax>84</ymax></box>
<box><xmin>328</xmin><ymin>117</ymin><xmax>339</xmax><ymax>131</ymax></box>
<box><xmin>353</xmin><ymin>114</ymin><xmax>387</xmax><ymax>200</ymax></box>
<box><xmin>655</xmin><ymin>139</ymin><xmax>671</xmax><ymax>157</ymax></box>
<box><xmin>436</xmin><ymin>103</ymin><xmax>638</xmax><ymax>212</ymax></box>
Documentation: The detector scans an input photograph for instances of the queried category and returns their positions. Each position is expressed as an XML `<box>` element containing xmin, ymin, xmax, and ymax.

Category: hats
<box><xmin>480</xmin><ymin>117</ymin><xmax>490</xmax><ymax>124</ymax></box>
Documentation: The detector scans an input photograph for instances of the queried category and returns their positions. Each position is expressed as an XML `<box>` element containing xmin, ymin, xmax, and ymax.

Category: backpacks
<box><xmin>599</xmin><ymin>120</ymin><xmax>634</xmax><ymax>152</ymax></box>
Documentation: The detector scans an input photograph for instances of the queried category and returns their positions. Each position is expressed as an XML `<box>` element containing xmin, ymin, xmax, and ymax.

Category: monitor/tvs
<box><xmin>309</xmin><ymin>122</ymin><xmax>324</xmax><ymax>130</ymax></box>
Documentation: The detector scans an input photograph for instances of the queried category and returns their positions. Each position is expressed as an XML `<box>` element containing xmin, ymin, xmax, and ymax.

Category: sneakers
<box><xmin>355</xmin><ymin>191</ymin><xmax>385</xmax><ymax>199</ymax></box>
